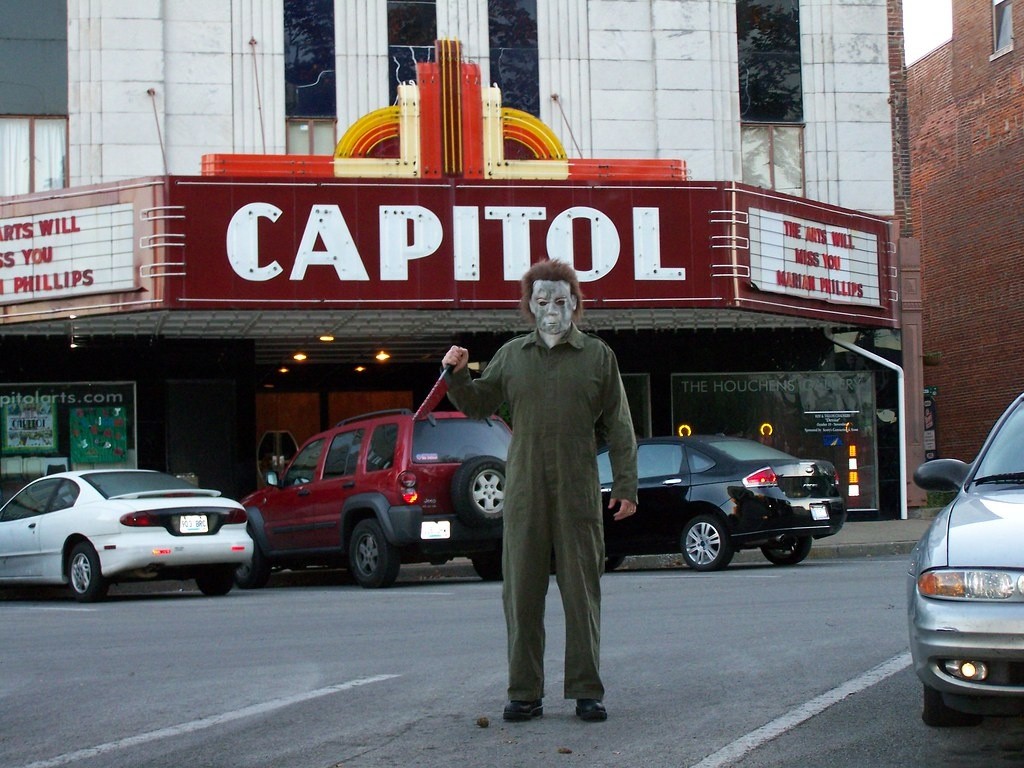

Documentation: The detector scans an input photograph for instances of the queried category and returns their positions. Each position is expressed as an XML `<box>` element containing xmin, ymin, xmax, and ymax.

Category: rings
<box><xmin>630</xmin><ymin>510</ymin><xmax>634</xmax><ymax>513</ymax></box>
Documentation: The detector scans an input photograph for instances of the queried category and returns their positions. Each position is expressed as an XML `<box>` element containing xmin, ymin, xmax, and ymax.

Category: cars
<box><xmin>0</xmin><ymin>468</ymin><xmax>254</xmax><ymax>603</ymax></box>
<box><xmin>903</xmin><ymin>392</ymin><xmax>1024</xmax><ymax>726</ymax></box>
<box><xmin>553</xmin><ymin>433</ymin><xmax>849</xmax><ymax>572</ymax></box>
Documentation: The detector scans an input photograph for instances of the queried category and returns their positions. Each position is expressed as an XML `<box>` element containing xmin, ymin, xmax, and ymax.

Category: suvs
<box><xmin>232</xmin><ymin>408</ymin><xmax>513</xmax><ymax>591</ymax></box>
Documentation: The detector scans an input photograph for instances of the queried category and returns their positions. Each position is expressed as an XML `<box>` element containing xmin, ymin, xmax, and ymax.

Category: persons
<box><xmin>442</xmin><ymin>261</ymin><xmax>642</xmax><ymax>722</ymax></box>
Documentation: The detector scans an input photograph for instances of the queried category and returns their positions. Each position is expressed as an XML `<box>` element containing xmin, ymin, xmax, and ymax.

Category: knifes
<box><xmin>413</xmin><ymin>351</ymin><xmax>462</xmax><ymax>420</ymax></box>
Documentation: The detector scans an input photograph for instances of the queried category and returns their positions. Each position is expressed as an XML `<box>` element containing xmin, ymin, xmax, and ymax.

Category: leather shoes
<box><xmin>576</xmin><ymin>699</ymin><xmax>607</xmax><ymax>720</ymax></box>
<box><xmin>503</xmin><ymin>698</ymin><xmax>544</xmax><ymax>720</ymax></box>
